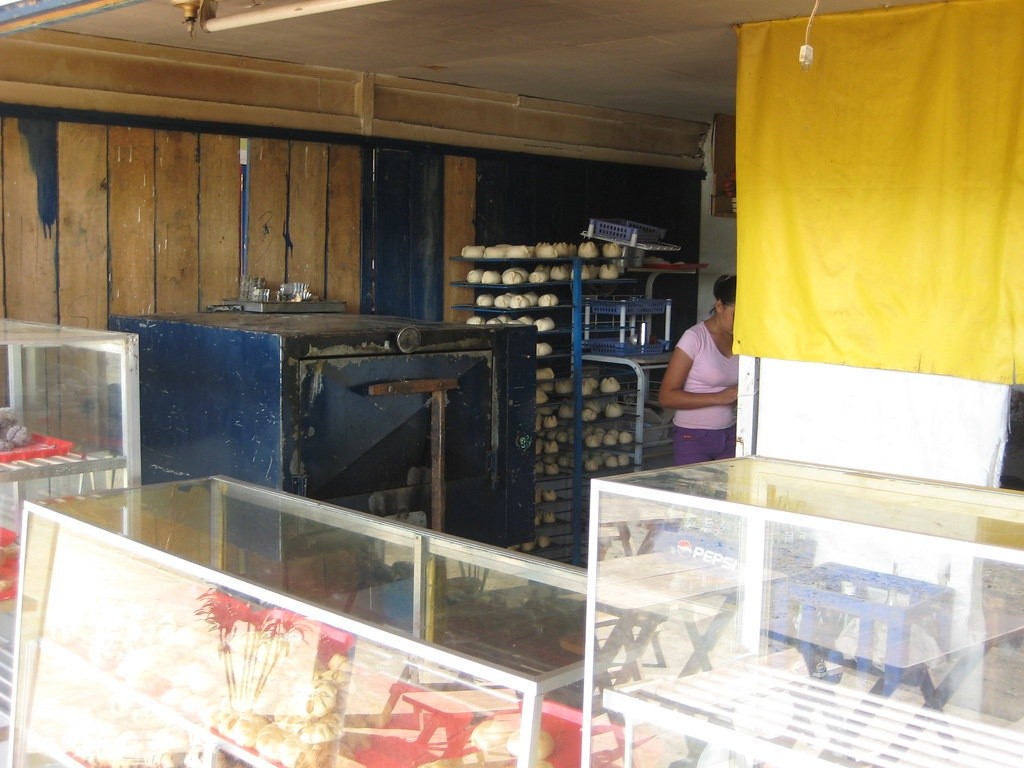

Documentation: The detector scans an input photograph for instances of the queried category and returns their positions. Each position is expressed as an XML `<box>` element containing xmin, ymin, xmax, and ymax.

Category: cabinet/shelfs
<box><xmin>0</xmin><ymin>318</ymin><xmax>141</xmax><ymax>768</ymax></box>
<box><xmin>8</xmin><ymin>476</ymin><xmax>723</xmax><ymax>768</ymax></box>
<box><xmin>580</xmin><ymin>456</ymin><xmax>1024</xmax><ymax>768</ymax></box>
<box><xmin>448</xmin><ymin>229</ymin><xmax>707</xmax><ymax>569</ymax></box>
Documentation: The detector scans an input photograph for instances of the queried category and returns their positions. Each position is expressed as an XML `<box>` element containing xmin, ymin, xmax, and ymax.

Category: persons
<box><xmin>658</xmin><ymin>276</ymin><xmax>739</xmax><ymax>467</ymax></box>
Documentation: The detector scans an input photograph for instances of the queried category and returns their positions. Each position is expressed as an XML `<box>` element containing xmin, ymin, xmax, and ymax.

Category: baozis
<box><xmin>31</xmin><ymin>591</ymin><xmax>556</xmax><ymax>768</ymax></box>
<box><xmin>461</xmin><ymin>241</ymin><xmax>632</xmax><ymax>552</ymax></box>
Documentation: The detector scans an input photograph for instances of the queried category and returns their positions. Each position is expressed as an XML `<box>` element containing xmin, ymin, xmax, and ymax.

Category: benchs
<box><xmin>374</xmin><ymin>502</ymin><xmax>1024</xmax><ymax>768</ymax></box>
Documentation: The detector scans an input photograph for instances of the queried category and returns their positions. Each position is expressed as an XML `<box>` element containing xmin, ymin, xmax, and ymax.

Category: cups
<box><xmin>254</xmin><ymin>289</ymin><xmax>270</xmax><ymax>304</ymax></box>
<box><xmin>280</xmin><ymin>282</ymin><xmax>304</xmax><ymax>303</ymax></box>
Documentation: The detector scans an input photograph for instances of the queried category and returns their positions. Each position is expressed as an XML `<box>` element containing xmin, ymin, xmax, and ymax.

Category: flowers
<box><xmin>723</xmin><ymin>172</ymin><xmax>736</xmax><ymax>201</ymax></box>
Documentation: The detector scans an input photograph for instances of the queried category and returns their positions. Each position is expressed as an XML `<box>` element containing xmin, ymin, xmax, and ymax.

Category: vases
<box><xmin>731</xmin><ymin>197</ymin><xmax>736</xmax><ymax>213</ymax></box>
